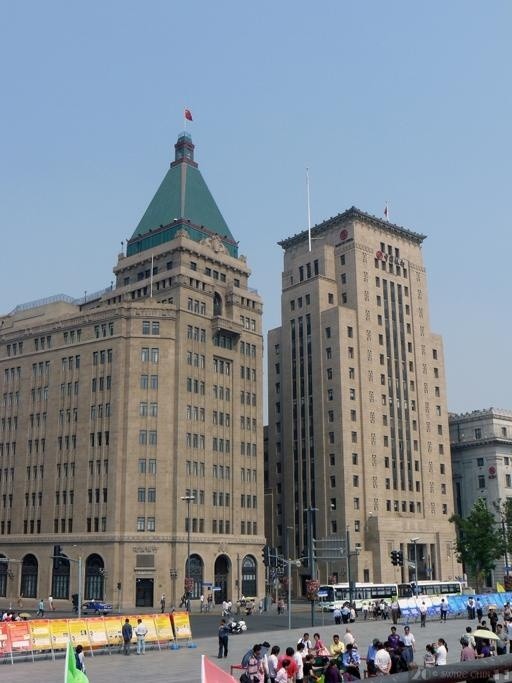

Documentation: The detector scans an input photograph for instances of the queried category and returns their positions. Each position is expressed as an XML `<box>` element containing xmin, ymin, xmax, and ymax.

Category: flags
<box><xmin>184</xmin><ymin>109</ymin><xmax>192</xmax><ymax>121</ymax></box>
<box><xmin>384</xmin><ymin>207</ymin><xmax>387</xmax><ymax>217</ymax></box>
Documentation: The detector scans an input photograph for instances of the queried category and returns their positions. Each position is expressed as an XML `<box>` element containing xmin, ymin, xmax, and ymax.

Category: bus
<box><xmin>398</xmin><ymin>579</ymin><xmax>462</xmax><ymax>599</ymax></box>
<box><xmin>318</xmin><ymin>581</ymin><xmax>397</xmax><ymax>612</ymax></box>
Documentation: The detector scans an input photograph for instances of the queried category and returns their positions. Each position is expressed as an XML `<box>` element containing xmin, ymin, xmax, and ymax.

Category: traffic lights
<box><xmin>269</xmin><ymin>549</ymin><xmax>277</xmax><ymax>566</ymax></box>
<box><xmin>262</xmin><ymin>545</ymin><xmax>269</xmax><ymax>565</ymax></box>
<box><xmin>398</xmin><ymin>551</ymin><xmax>403</xmax><ymax>567</ymax></box>
<box><xmin>390</xmin><ymin>551</ymin><xmax>397</xmax><ymax>565</ymax></box>
<box><xmin>117</xmin><ymin>582</ymin><xmax>121</xmax><ymax>590</ymax></box>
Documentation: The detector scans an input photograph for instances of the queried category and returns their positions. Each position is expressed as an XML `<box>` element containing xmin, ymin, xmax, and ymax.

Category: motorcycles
<box><xmin>225</xmin><ymin>615</ymin><xmax>249</xmax><ymax>633</ymax></box>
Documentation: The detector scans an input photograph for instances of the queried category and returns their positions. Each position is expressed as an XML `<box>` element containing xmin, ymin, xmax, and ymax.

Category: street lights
<box><xmin>302</xmin><ymin>503</ymin><xmax>320</xmax><ymax>582</ymax></box>
<box><xmin>500</xmin><ymin>517</ymin><xmax>509</xmax><ymax>580</ymax></box>
<box><xmin>181</xmin><ymin>494</ymin><xmax>197</xmax><ymax>610</ymax></box>
<box><xmin>339</xmin><ymin>523</ymin><xmax>363</xmax><ymax>610</ymax></box>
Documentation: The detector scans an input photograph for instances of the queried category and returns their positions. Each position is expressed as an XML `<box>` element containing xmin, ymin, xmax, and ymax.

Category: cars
<box><xmin>72</xmin><ymin>599</ymin><xmax>113</xmax><ymax>615</ymax></box>
